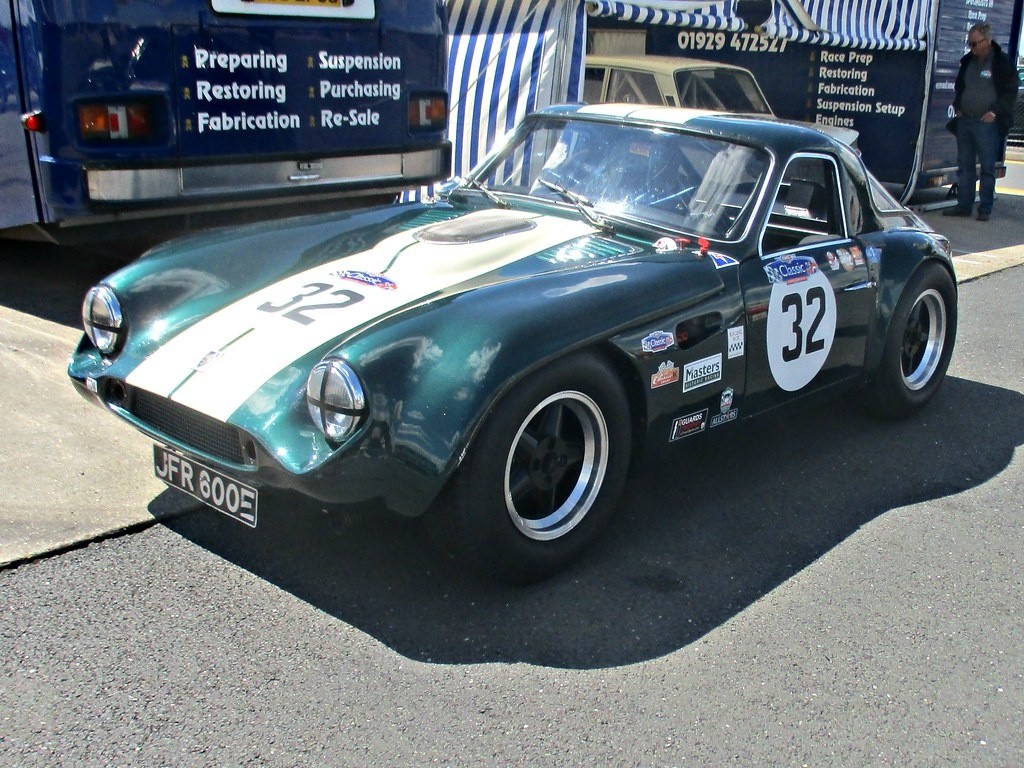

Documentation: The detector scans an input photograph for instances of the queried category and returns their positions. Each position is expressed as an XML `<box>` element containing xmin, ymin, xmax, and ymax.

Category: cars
<box><xmin>67</xmin><ymin>102</ymin><xmax>958</xmax><ymax>585</ymax></box>
<box><xmin>583</xmin><ymin>55</ymin><xmax>862</xmax><ymax>185</ymax></box>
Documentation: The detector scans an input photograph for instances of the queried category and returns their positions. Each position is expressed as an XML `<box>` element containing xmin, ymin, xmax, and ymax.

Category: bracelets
<box><xmin>989</xmin><ymin>111</ymin><xmax>996</xmax><ymax>117</ymax></box>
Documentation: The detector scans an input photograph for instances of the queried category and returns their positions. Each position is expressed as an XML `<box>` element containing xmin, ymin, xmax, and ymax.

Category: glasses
<box><xmin>967</xmin><ymin>39</ymin><xmax>984</xmax><ymax>47</ymax></box>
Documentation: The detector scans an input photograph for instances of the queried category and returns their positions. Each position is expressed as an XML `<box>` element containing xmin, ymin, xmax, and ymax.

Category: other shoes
<box><xmin>943</xmin><ymin>206</ymin><xmax>971</xmax><ymax>216</ymax></box>
<box><xmin>977</xmin><ymin>212</ymin><xmax>989</xmax><ymax>220</ymax></box>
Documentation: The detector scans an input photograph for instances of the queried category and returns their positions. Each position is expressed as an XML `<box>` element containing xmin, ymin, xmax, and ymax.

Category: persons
<box><xmin>941</xmin><ymin>22</ymin><xmax>1019</xmax><ymax>221</ymax></box>
